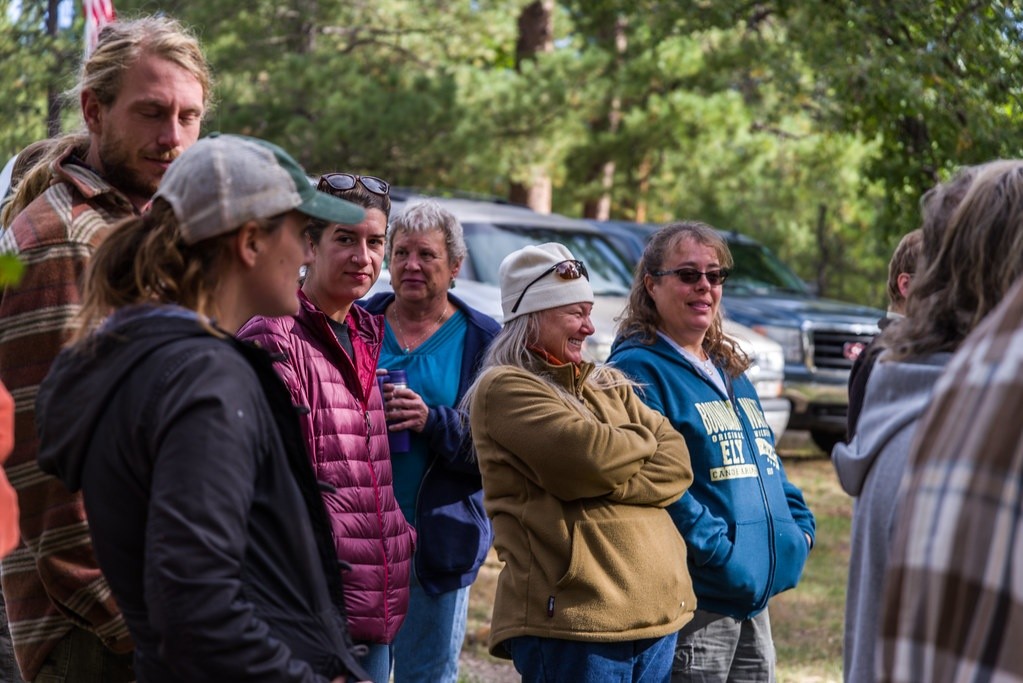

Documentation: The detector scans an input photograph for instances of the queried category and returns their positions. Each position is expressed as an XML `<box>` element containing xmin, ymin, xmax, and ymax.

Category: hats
<box><xmin>158</xmin><ymin>130</ymin><xmax>364</xmax><ymax>243</ymax></box>
<box><xmin>499</xmin><ymin>242</ymin><xmax>594</xmax><ymax>324</ymax></box>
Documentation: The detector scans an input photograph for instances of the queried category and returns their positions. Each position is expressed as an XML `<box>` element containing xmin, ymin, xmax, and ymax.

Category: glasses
<box><xmin>317</xmin><ymin>173</ymin><xmax>391</xmax><ymax>196</ymax></box>
<box><xmin>654</xmin><ymin>268</ymin><xmax>728</xmax><ymax>284</ymax></box>
<box><xmin>511</xmin><ymin>260</ymin><xmax>589</xmax><ymax>313</ymax></box>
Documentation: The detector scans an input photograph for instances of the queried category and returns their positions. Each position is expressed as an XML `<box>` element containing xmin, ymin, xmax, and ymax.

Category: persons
<box><xmin>354</xmin><ymin>198</ymin><xmax>503</xmax><ymax>683</ymax></box>
<box><xmin>468</xmin><ymin>241</ymin><xmax>697</xmax><ymax>683</ymax></box>
<box><xmin>605</xmin><ymin>222</ymin><xmax>816</xmax><ymax>683</ymax></box>
<box><xmin>0</xmin><ymin>20</ymin><xmax>212</xmax><ymax>683</ymax></box>
<box><xmin>236</xmin><ymin>175</ymin><xmax>418</xmax><ymax>682</ymax></box>
<box><xmin>830</xmin><ymin>161</ymin><xmax>1023</xmax><ymax>683</ymax></box>
<box><xmin>34</xmin><ymin>132</ymin><xmax>364</xmax><ymax>682</ymax></box>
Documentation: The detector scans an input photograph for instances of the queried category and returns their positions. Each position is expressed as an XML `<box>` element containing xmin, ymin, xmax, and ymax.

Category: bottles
<box><xmin>377</xmin><ymin>370</ymin><xmax>416</xmax><ymax>454</ymax></box>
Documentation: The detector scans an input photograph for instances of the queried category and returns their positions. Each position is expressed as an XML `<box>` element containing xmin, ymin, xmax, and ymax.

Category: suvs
<box><xmin>376</xmin><ymin>185</ymin><xmax>790</xmax><ymax>469</ymax></box>
<box><xmin>600</xmin><ymin>219</ymin><xmax>903</xmax><ymax>466</ymax></box>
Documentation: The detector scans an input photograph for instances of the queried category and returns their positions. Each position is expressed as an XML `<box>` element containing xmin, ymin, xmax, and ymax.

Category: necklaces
<box><xmin>392</xmin><ymin>302</ymin><xmax>448</xmax><ymax>355</ymax></box>
<box><xmin>664</xmin><ymin>327</ymin><xmax>713</xmax><ymax>376</ymax></box>
